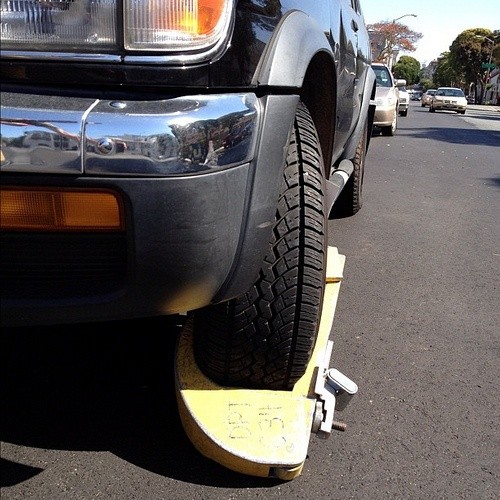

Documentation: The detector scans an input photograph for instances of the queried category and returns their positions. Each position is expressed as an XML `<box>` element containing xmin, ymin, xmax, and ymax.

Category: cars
<box><xmin>422</xmin><ymin>90</ymin><xmax>437</xmax><ymax>107</ymax></box>
<box><xmin>0</xmin><ymin>0</ymin><xmax>378</xmax><ymax>391</ymax></box>
<box><xmin>394</xmin><ymin>79</ymin><xmax>409</xmax><ymax>116</ymax></box>
<box><xmin>370</xmin><ymin>62</ymin><xmax>406</xmax><ymax>137</ymax></box>
<box><xmin>406</xmin><ymin>89</ymin><xmax>423</xmax><ymax>101</ymax></box>
<box><xmin>430</xmin><ymin>87</ymin><xmax>467</xmax><ymax>114</ymax></box>
